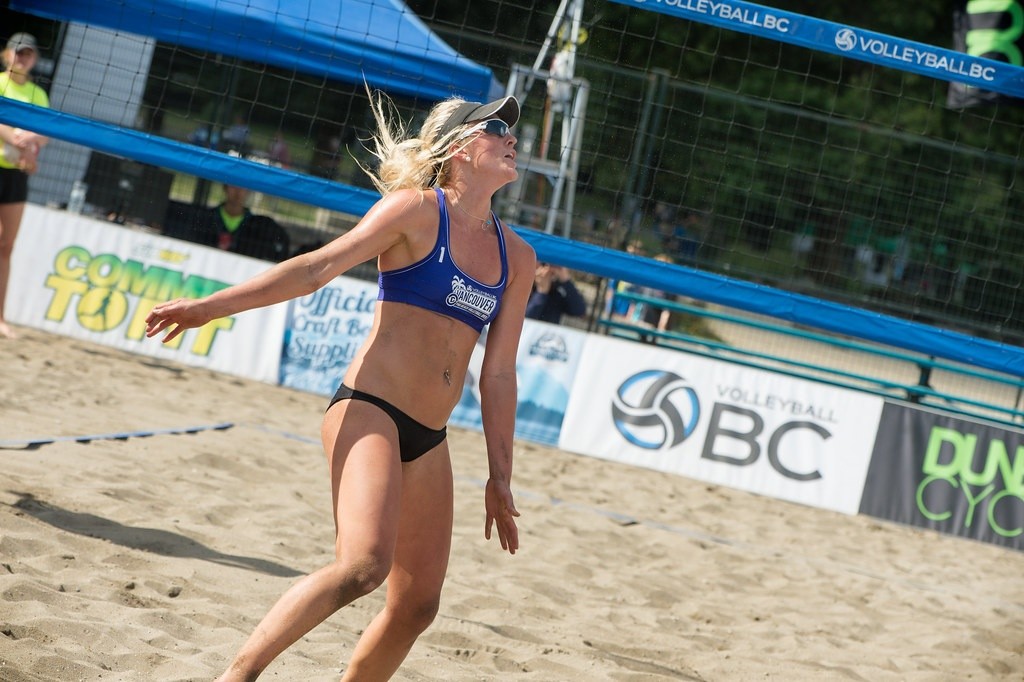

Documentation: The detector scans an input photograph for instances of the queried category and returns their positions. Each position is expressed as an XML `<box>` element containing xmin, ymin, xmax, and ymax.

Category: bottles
<box><xmin>107</xmin><ymin>179</ymin><xmax>135</xmax><ymax>224</ymax></box>
<box><xmin>66</xmin><ymin>179</ymin><xmax>89</xmax><ymax>215</ymax></box>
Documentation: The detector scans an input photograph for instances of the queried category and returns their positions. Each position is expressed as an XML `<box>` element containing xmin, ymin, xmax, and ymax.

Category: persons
<box><xmin>146</xmin><ymin>75</ymin><xmax>536</xmax><ymax>682</ymax></box>
<box><xmin>0</xmin><ymin>35</ymin><xmax>50</xmax><ymax>339</ymax></box>
<box><xmin>142</xmin><ymin>92</ymin><xmax>1024</xmax><ymax>347</ymax></box>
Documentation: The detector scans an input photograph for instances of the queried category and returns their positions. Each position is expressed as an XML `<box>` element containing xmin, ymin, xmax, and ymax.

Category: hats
<box><xmin>8</xmin><ymin>33</ymin><xmax>38</xmax><ymax>53</ymax></box>
<box><xmin>435</xmin><ymin>96</ymin><xmax>520</xmax><ymax>163</ymax></box>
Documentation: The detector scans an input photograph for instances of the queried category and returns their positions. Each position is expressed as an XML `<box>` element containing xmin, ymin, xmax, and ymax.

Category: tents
<box><xmin>0</xmin><ymin>0</ymin><xmax>492</xmax><ymax>199</ymax></box>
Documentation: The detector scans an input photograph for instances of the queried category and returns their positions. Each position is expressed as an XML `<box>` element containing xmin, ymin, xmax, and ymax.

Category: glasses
<box><xmin>467</xmin><ymin>119</ymin><xmax>510</xmax><ymax>137</ymax></box>
